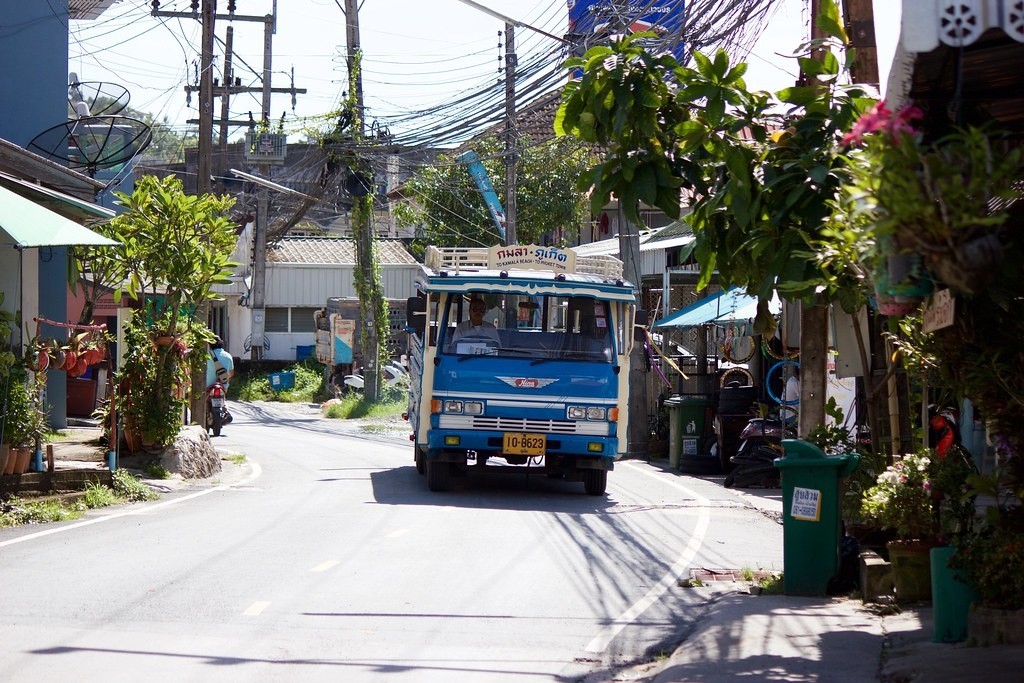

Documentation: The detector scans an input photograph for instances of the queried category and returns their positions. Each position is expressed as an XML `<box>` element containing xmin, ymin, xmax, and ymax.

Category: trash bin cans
<box><xmin>772</xmin><ymin>438</ymin><xmax>862</xmax><ymax>598</ymax></box>
<box><xmin>662</xmin><ymin>393</ymin><xmax>708</xmax><ymax>470</ymax></box>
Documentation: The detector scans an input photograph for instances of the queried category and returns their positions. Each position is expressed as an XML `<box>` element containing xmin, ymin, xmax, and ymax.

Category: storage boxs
<box><xmin>268</xmin><ymin>371</ymin><xmax>297</xmax><ymax>390</ymax></box>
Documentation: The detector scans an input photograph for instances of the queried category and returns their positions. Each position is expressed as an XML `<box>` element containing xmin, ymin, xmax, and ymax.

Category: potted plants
<box><xmin>0</xmin><ymin>379</ymin><xmax>54</xmax><ymax>475</ymax></box>
<box><xmin>648</xmin><ymin>394</ymin><xmax>671</xmax><ymax>458</ymax></box>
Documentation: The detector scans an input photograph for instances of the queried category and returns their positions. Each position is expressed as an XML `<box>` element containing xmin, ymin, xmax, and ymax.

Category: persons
<box><xmin>781</xmin><ymin>368</ymin><xmax>799</xmax><ymax>423</ymax></box>
<box><xmin>929</xmin><ymin>403</ymin><xmax>962</xmax><ymax>460</ymax></box>
<box><xmin>206</xmin><ymin>333</ymin><xmax>234</xmax><ymax>395</ymax></box>
<box><xmin>451</xmin><ymin>298</ymin><xmax>503</xmax><ymax>348</ymax></box>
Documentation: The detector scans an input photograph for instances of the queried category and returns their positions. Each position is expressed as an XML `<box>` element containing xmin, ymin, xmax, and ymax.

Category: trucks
<box><xmin>404</xmin><ymin>241</ymin><xmax>650</xmax><ymax>496</ymax></box>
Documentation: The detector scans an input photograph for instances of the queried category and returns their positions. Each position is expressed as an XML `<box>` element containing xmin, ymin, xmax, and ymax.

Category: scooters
<box><xmin>729</xmin><ymin>415</ymin><xmax>794</xmax><ymax>487</ymax></box>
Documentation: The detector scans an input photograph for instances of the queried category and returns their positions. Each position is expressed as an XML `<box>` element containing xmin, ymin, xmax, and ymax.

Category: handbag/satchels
<box><xmin>216</xmin><ymin>368</ymin><xmax>228</xmax><ymax>384</ymax></box>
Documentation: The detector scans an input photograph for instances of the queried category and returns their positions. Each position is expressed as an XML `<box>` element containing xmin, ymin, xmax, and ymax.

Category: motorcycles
<box><xmin>205</xmin><ymin>383</ymin><xmax>233</xmax><ymax>438</ymax></box>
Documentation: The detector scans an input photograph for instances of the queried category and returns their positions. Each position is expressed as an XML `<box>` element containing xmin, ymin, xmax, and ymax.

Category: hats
<box><xmin>213</xmin><ymin>334</ymin><xmax>221</xmax><ymax>341</ymax></box>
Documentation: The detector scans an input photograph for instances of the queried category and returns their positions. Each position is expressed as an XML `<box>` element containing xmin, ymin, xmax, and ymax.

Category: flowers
<box><xmin>858</xmin><ymin>452</ymin><xmax>977</xmax><ymax>544</ymax></box>
<box><xmin>948</xmin><ymin>432</ymin><xmax>1024</xmax><ymax>610</ymax></box>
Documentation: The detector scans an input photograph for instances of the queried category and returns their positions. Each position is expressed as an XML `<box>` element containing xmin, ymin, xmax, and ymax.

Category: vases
<box><xmin>985</xmin><ymin>606</ymin><xmax>1024</xmax><ymax>643</ymax></box>
<box><xmin>885</xmin><ymin>540</ymin><xmax>931</xmax><ymax>604</ymax></box>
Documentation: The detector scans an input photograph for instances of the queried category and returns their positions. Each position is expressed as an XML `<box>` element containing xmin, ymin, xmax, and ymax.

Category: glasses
<box><xmin>470</xmin><ymin>307</ymin><xmax>486</xmax><ymax>312</ymax></box>
